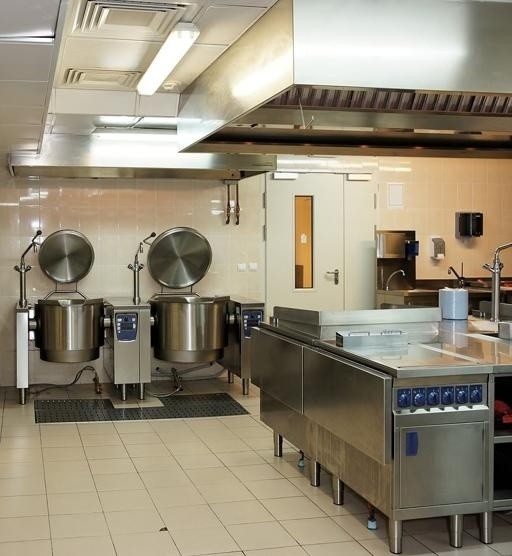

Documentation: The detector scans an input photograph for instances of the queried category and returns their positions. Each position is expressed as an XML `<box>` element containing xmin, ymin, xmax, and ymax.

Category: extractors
<box><xmin>176</xmin><ymin>2</ymin><xmax>512</xmax><ymax>159</ymax></box>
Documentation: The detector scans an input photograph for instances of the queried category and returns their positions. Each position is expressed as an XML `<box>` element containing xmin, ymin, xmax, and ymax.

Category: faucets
<box><xmin>447</xmin><ymin>266</ymin><xmax>469</xmax><ymax>288</ymax></box>
<box><xmin>482</xmin><ymin>243</ymin><xmax>511</xmax><ymax>322</ymax></box>
<box><xmin>384</xmin><ymin>268</ymin><xmax>407</xmax><ymax>292</ymax></box>
